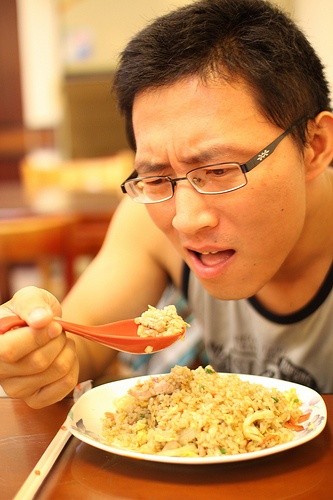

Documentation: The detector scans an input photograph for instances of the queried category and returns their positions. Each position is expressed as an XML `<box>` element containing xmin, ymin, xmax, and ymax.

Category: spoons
<box><xmin>1</xmin><ymin>315</ymin><xmax>187</xmax><ymax>353</ymax></box>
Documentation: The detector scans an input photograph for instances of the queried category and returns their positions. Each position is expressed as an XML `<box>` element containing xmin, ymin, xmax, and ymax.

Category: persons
<box><xmin>1</xmin><ymin>0</ymin><xmax>331</xmax><ymax>409</ymax></box>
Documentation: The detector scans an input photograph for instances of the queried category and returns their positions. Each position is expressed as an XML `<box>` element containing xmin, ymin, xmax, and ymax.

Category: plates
<box><xmin>66</xmin><ymin>372</ymin><xmax>329</xmax><ymax>464</ymax></box>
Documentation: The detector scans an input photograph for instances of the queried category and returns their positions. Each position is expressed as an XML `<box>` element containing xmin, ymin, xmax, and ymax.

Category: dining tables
<box><xmin>0</xmin><ymin>395</ymin><xmax>333</xmax><ymax>500</ymax></box>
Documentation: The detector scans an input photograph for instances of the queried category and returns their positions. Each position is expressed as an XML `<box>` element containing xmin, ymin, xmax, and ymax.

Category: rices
<box><xmin>104</xmin><ymin>303</ymin><xmax>303</xmax><ymax>455</ymax></box>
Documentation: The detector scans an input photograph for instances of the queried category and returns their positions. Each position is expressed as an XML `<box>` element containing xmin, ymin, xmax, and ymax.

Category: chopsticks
<box><xmin>12</xmin><ymin>419</ymin><xmax>73</xmax><ymax>500</ymax></box>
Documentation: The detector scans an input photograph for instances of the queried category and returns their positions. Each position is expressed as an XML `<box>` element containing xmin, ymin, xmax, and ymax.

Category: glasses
<box><xmin>120</xmin><ymin>114</ymin><xmax>313</xmax><ymax>204</ymax></box>
